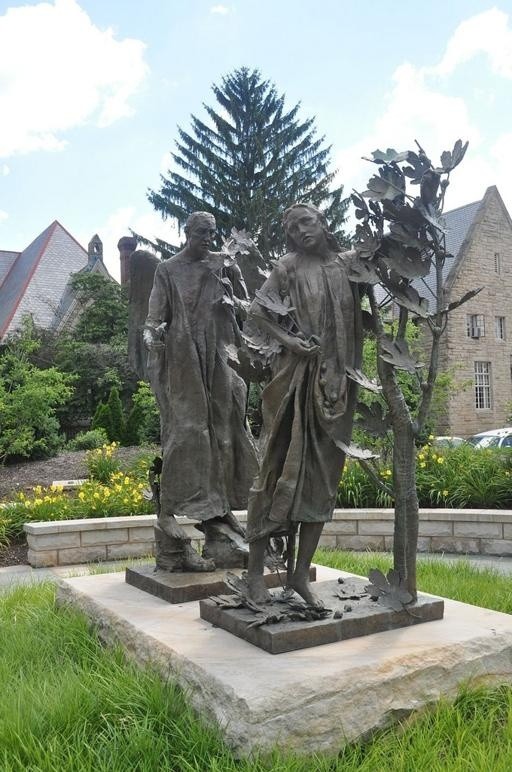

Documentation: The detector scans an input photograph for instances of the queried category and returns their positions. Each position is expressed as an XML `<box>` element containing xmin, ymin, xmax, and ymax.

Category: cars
<box><xmin>432</xmin><ymin>428</ymin><xmax>511</xmax><ymax>449</ymax></box>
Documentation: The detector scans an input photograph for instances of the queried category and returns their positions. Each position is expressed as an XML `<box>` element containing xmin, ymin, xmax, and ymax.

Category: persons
<box><xmin>244</xmin><ymin>168</ymin><xmax>440</xmax><ymax>607</ymax></box>
<box><xmin>143</xmin><ymin>211</ymin><xmax>250</xmax><ymax>540</ymax></box>
